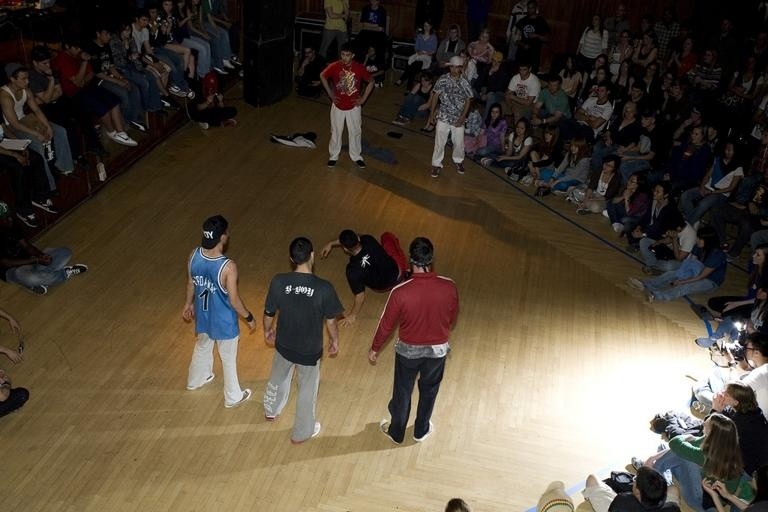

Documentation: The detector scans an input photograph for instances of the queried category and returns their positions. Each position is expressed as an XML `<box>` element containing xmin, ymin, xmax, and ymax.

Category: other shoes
<box><xmin>696</xmin><ymin>338</ymin><xmax>718</xmax><ymax>348</ymax></box>
<box><xmin>714</xmin><ymin>317</ymin><xmax>724</xmax><ymax>323</ymax></box>
<box><xmin>624</xmin><ymin>276</ymin><xmax>656</xmax><ymax>304</ymax></box>
<box><xmin>478</xmin><ymin>157</ymin><xmax>592</xmax><ymax>216</ymax></box>
<box><xmin>602</xmin><ymin>210</ymin><xmax>661</xmax><ymax>275</ymax></box>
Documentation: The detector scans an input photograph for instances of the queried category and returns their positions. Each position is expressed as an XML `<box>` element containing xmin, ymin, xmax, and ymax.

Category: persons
<box><xmin>1</xmin><ymin>310</ymin><xmax>29</xmax><ymax>418</ymax></box>
<box><xmin>446</xmin><ymin>333</ymin><xmax>768</xmax><ymax>511</ymax></box>
<box><xmin>295</xmin><ymin>0</ymin><xmax>392</xmax><ymax>169</ymax></box>
<box><xmin>1</xmin><ymin>0</ymin><xmax>242</xmax><ymax>296</ymax></box>
<box><xmin>393</xmin><ymin>0</ymin><xmax>767</xmax><ymax>347</ymax></box>
<box><xmin>181</xmin><ymin>214</ymin><xmax>459</xmax><ymax>444</ymax></box>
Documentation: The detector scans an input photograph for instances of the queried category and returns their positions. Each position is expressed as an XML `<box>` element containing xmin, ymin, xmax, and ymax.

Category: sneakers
<box><xmin>412</xmin><ymin>420</ymin><xmax>434</xmax><ymax>442</ymax></box>
<box><xmin>379</xmin><ymin>419</ymin><xmax>405</xmax><ymax>445</ymax></box>
<box><xmin>64</xmin><ymin>263</ymin><xmax>89</xmax><ymax>280</ymax></box>
<box><xmin>265</xmin><ymin>413</ymin><xmax>276</xmax><ymax>420</ymax></box>
<box><xmin>24</xmin><ymin>284</ymin><xmax>48</xmax><ymax>296</ymax></box>
<box><xmin>356</xmin><ymin>159</ymin><xmax>366</xmax><ymax>169</ymax></box>
<box><xmin>455</xmin><ymin>163</ymin><xmax>465</xmax><ymax>174</ymax></box>
<box><xmin>430</xmin><ymin>166</ymin><xmax>441</xmax><ymax>178</ymax></box>
<box><xmin>391</xmin><ymin>114</ymin><xmax>436</xmax><ymax>133</ymax></box>
<box><xmin>128</xmin><ymin>56</ymin><xmax>242</xmax><ymax>131</ymax></box>
<box><xmin>0</xmin><ymin>386</ymin><xmax>30</xmax><ymax>418</ymax></box>
<box><xmin>15</xmin><ymin>130</ymin><xmax>138</xmax><ymax>227</ymax></box>
<box><xmin>327</xmin><ymin>159</ymin><xmax>337</xmax><ymax>168</ymax></box>
<box><xmin>224</xmin><ymin>388</ymin><xmax>251</xmax><ymax>409</ymax></box>
<box><xmin>290</xmin><ymin>421</ymin><xmax>321</xmax><ymax>443</ymax></box>
<box><xmin>186</xmin><ymin>372</ymin><xmax>215</xmax><ymax>391</ymax></box>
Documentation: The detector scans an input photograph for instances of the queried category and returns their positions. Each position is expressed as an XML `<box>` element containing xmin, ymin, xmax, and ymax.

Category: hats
<box><xmin>201</xmin><ymin>215</ymin><xmax>228</xmax><ymax>250</ymax></box>
<box><xmin>5</xmin><ymin>63</ymin><xmax>23</xmax><ymax>78</ymax></box>
<box><xmin>445</xmin><ymin>56</ymin><xmax>464</xmax><ymax>67</ymax></box>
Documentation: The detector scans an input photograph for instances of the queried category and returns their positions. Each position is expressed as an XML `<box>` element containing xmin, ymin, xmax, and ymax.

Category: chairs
<box><xmin>0</xmin><ymin>0</ymin><xmax>241</xmax><ymax>242</ymax></box>
